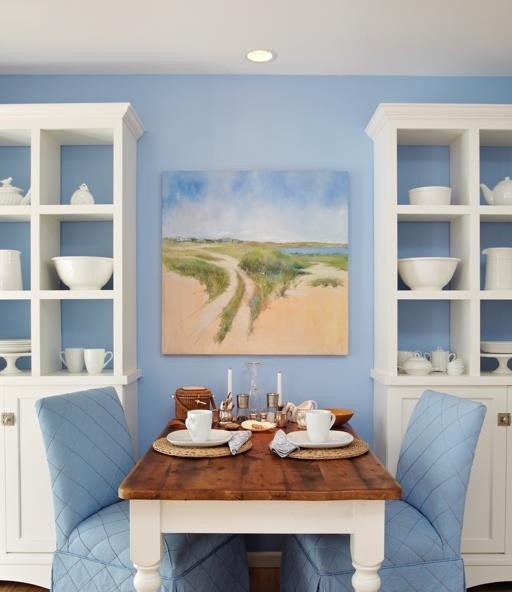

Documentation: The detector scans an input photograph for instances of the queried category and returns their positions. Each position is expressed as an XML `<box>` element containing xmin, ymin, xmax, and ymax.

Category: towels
<box><xmin>270</xmin><ymin>429</ymin><xmax>298</xmax><ymax>458</ymax></box>
<box><xmin>227</xmin><ymin>431</ymin><xmax>252</xmax><ymax>454</ymax></box>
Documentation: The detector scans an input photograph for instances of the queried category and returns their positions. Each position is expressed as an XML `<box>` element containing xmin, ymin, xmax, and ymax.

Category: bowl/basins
<box><xmin>49</xmin><ymin>256</ymin><xmax>112</xmax><ymax>291</ymax></box>
<box><xmin>397</xmin><ymin>257</ymin><xmax>460</xmax><ymax>292</ymax></box>
<box><xmin>479</xmin><ymin>340</ymin><xmax>511</xmax><ymax>354</ymax></box>
<box><xmin>407</xmin><ymin>186</ymin><xmax>451</xmax><ymax>205</ymax></box>
<box><xmin>318</xmin><ymin>407</ymin><xmax>354</xmax><ymax>430</ymax></box>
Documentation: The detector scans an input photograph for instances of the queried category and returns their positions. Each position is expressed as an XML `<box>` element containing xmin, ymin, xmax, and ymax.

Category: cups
<box><xmin>306</xmin><ymin>409</ymin><xmax>336</xmax><ymax>443</ymax></box>
<box><xmin>57</xmin><ymin>347</ymin><xmax>84</xmax><ymax>374</ymax></box>
<box><xmin>481</xmin><ymin>247</ymin><xmax>512</xmax><ymax>291</ymax></box>
<box><xmin>83</xmin><ymin>348</ymin><xmax>114</xmax><ymax>373</ymax></box>
<box><xmin>184</xmin><ymin>409</ymin><xmax>212</xmax><ymax>441</ymax></box>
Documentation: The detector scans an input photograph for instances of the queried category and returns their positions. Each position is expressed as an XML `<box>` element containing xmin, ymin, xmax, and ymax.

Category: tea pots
<box><xmin>397</xmin><ymin>346</ymin><xmax>466</xmax><ymax>375</ymax></box>
<box><xmin>1</xmin><ymin>177</ymin><xmax>31</xmax><ymax>206</ymax></box>
<box><xmin>479</xmin><ymin>177</ymin><xmax>512</xmax><ymax>207</ymax></box>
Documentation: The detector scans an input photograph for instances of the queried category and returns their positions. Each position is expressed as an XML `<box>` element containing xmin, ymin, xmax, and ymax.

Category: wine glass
<box><xmin>245</xmin><ymin>361</ymin><xmax>267</xmax><ymax>422</ymax></box>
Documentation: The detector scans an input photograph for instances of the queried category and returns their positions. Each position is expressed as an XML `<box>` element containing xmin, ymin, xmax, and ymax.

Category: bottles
<box><xmin>295</xmin><ymin>408</ymin><xmax>306</xmax><ymax>429</ymax></box>
<box><xmin>219</xmin><ymin>392</ymin><xmax>289</xmax><ymax>428</ymax></box>
<box><xmin>70</xmin><ymin>183</ymin><xmax>95</xmax><ymax>206</ymax></box>
<box><xmin>0</xmin><ymin>249</ymin><xmax>24</xmax><ymax>291</ymax></box>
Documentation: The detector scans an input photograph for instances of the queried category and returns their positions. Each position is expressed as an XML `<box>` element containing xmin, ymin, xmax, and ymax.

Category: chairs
<box><xmin>279</xmin><ymin>387</ymin><xmax>484</xmax><ymax>589</ymax></box>
<box><xmin>31</xmin><ymin>388</ymin><xmax>248</xmax><ymax>591</ymax></box>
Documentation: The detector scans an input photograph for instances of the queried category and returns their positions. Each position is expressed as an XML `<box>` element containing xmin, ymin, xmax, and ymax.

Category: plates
<box><xmin>0</xmin><ymin>339</ymin><xmax>32</xmax><ymax>354</ymax></box>
<box><xmin>165</xmin><ymin>429</ymin><xmax>233</xmax><ymax>446</ymax></box>
<box><xmin>240</xmin><ymin>419</ymin><xmax>277</xmax><ymax>432</ymax></box>
<box><xmin>285</xmin><ymin>431</ymin><xmax>353</xmax><ymax>449</ymax></box>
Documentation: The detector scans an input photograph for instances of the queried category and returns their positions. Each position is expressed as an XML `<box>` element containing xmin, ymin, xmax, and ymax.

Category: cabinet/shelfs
<box><xmin>1</xmin><ymin>100</ymin><xmax>146</xmax><ymax>592</ymax></box>
<box><xmin>365</xmin><ymin>102</ymin><xmax>512</xmax><ymax>589</ymax></box>
